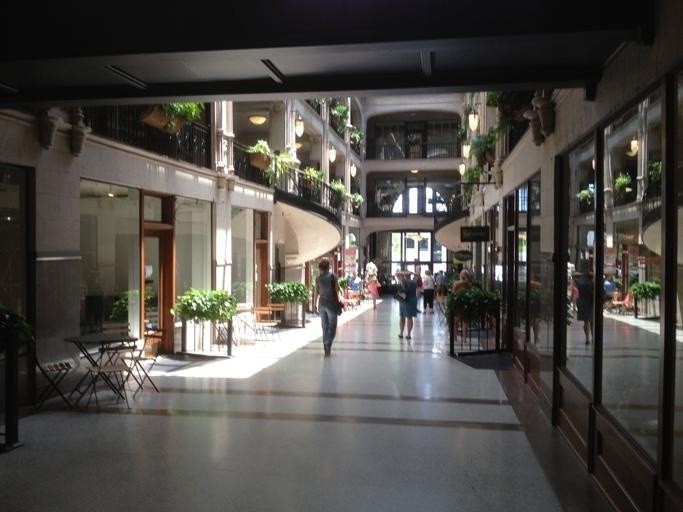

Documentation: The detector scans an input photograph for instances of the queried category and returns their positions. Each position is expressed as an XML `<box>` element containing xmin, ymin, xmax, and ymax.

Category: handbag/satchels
<box><xmin>335</xmin><ymin>294</ymin><xmax>343</xmax><ymax>315</ymax></box>
<box><xmin>394</xmin><ymin>290</ymin><xmax>408</xmax><ymax>302</ymax></box>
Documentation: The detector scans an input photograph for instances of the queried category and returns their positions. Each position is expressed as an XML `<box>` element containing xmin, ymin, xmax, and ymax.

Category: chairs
<box><xmin>27</xmin><ymin>325</ymin><xmax>167</xmax><ymax>416</ymax></box>
<box><xmin>237</xmin><ymin>299</ymin><xmax>286</xmax><ymax>344</ymax></box>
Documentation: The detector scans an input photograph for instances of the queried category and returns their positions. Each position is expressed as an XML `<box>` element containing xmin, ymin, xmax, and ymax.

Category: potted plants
<box><xmin>140</xmin><ymin>100</ymin><xmax>205</xmax><ymax>137</ymax></box>
<box><xmin>246</xmin><ymin>138</ymin><xmax>350</xmax><ymax>205</ymax></box>
<box><xmin>451</xmin><ymin>124</ymin><xmax>498</xmax><ymax>191</ymax></box>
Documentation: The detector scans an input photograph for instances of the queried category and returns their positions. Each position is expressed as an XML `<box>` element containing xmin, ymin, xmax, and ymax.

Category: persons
<box><xmin>572</xmin><ymin>260</ymin><xmax>594</xmax><ymax>344</ymax></box>
<box><xmin>395</xmin><ymin>263</ymin><xmax>463</xmax><ymax>339</ymax></box>
<box><xmin>348</xmin><ymin>271</ymin><xmax>361</xmax><ymax>290</ymax></box>
<box><xmin>313</xmin><ymin>261</ymin><xmax>340</xmax><ymax>358</ymax></box>
<box><xmin>365</xmin><ymin>272</ymin><xmax>379</xmax><ymax>310</ymax></box>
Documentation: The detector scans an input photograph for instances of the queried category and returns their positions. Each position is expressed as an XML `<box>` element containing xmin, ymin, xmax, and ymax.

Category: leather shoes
<box><xmin>399</xmin><ymin>336</ymin><xmax>411</xmax><ymax>339</ymax></box>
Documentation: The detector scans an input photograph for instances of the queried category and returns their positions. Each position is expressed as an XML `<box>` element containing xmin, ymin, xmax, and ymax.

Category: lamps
<box><xmin>246</xmin><ymin>113</ymin><xmax>267</xmax><ymax>127</ymax></box>
